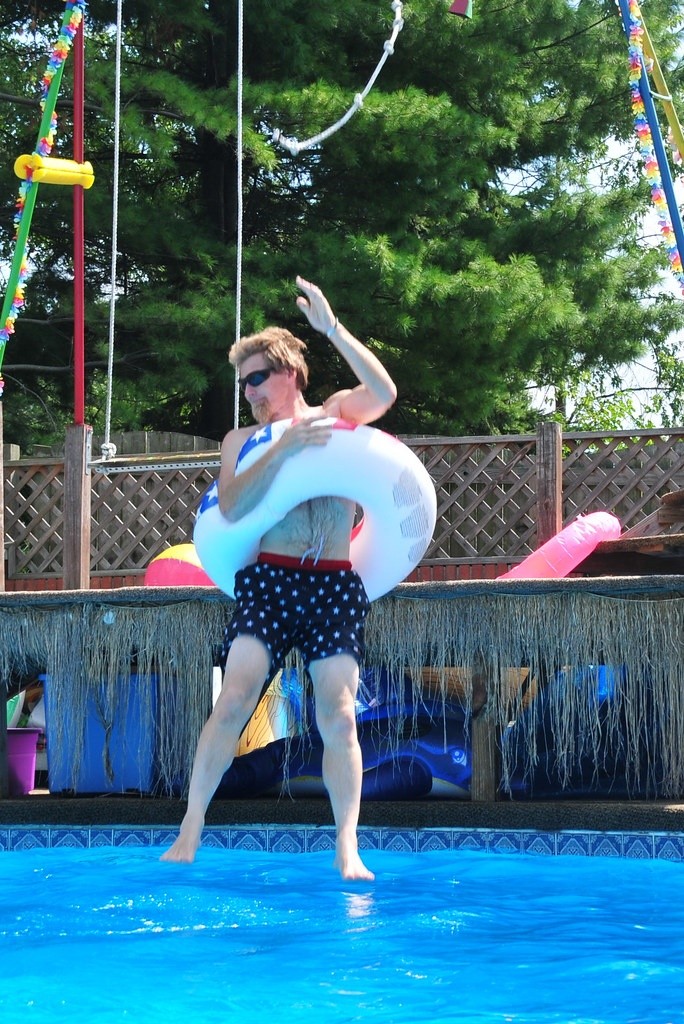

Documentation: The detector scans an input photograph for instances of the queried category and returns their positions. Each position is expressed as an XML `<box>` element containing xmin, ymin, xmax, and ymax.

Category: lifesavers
<box><xmin>192</xmin><ymin>412</ymin><xmax>442</xmax><ymax>607</ymax></box>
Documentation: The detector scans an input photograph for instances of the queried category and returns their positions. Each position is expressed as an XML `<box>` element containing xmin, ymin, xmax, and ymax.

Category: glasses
<box><xmin>238</xmin><ymin>368</ymin><xmax>274</xmax><ymax>392</ymax></box>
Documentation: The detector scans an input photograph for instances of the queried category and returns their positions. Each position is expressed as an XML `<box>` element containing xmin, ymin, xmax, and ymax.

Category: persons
<box><xmin>157</xmin><ymin>274</ymin><xmax>398</xmax><ymax>882</ymax></box>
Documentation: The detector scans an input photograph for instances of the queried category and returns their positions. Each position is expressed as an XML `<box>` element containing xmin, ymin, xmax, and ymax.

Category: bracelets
<box><xmin>325</xmin><ymin>315</ymin><xmax>339</xmax><ymax>339</ymax></box>
